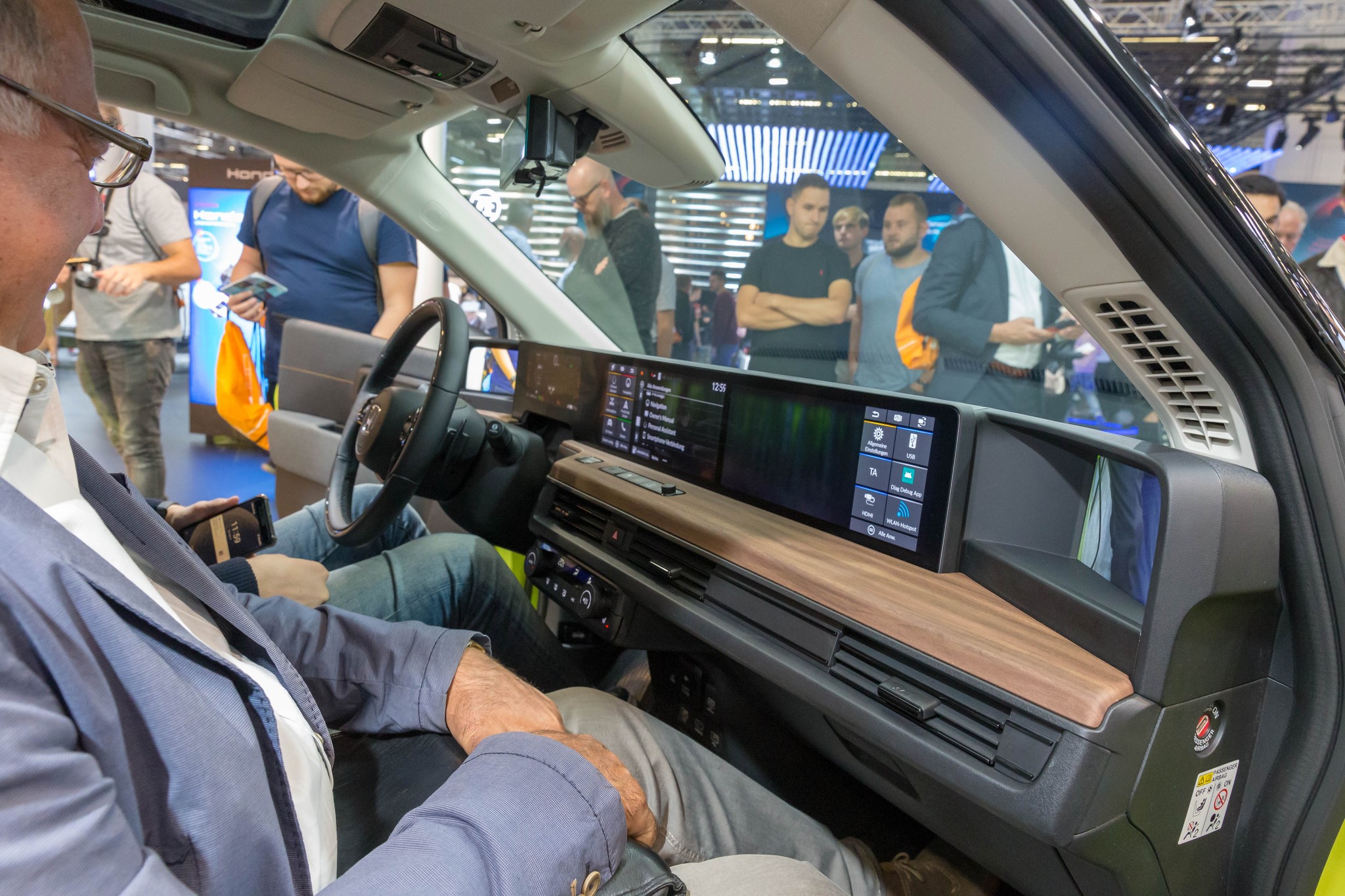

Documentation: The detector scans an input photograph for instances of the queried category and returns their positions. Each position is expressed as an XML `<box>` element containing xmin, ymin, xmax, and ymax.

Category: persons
<box><xmin>909</xmin><ymin>212</ymin><xmax>1084</xmax><ymax>421</ymax></box>
<box><xmin>734</xmin><ymin>172</ymin><xmax>854</xmax><ymax>382</ymax></box>
<box><xmin>567</xmin><ymin>155</ymin><xmax>662</xmax><ymax>358</ymax></box>
<box><xmin>849</xmin><ymin>193</ymin><xmax>941</xmax><ymax>402</ymax></box>
<box><xmin>36</xmin><ymin>100</ymin><xmax>205</xmax><ymax>502</ymax></box>
<box><xmin>1047</xmin><ymin>302</ymin><xmax>1108</xmax><ymax>423</ymax></box>
<box><xmin>227</xmin><ymin>148</ymin><xmax>420</xmax><ymax>406</ymax></box>
<box><xmin>0</xmin><ymin>0</ymin><xmax>1002</xmax><ymax>895</ymax></box>
<box><xmin>622</xmin><ymin>194</ymin><xmax>747</xmax><ymax>370</ymax></box>
<box><xmin>829</xmin><ymin>204</ymin><xmax>871</xmax><ymax>320</ymax></box>
<box><xmin>100</xmin><ymin>467</ymin><xmax>659</xmax><ymax>710</ymax></box>
<box><xmin>448</xmin><ymin>199</ymin><xmax>587</xmax><ymax>398</ymax></box>
<box><xmin>1235</xmin><ymin>174</ymin><xmax>1345</xmax><ymax>330</ymax></box>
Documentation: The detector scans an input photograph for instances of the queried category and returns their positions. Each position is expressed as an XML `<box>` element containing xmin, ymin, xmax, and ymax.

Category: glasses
<box><xmin>0</xmin><ymin>75</ymin><xmax>153</xmax><ymax>186</ymax></box>
<box><xmin>568</xmin><ymin>182</ymin><xmax>601</xmax><ymax>208</ymax></box>
<box><xmin>269</xmin><ymin>157</ymin><xmax>324</xmax><ymax>182</ymax></box>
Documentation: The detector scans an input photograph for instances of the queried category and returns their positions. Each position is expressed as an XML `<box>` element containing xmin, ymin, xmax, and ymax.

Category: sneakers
<box><xmin>845</xmin><ymin>839</ymin><xmax>980</xmax><ymax>896</ymax></box>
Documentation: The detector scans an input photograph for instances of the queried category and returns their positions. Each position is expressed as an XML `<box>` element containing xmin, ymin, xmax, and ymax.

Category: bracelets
<box><xmin>916</xmin><ymin>377</ymin><xmax>929</xmax><ymax>391</ymax></box>
<box><xmin>508</xmin><ymin>375</ymin><xmax>517</xmax><ymax>386</ymax></box>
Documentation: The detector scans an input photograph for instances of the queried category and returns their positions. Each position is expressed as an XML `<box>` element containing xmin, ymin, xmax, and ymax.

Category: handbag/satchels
<box><xmin>215</xmin><ymin>307</ymin><xmax>274</xmax><ymax>452</ymax></box>
<box><xmin>895</xmin><ymin>270</ymin><xmax>943</xmax><ymax>370</ymax></box>
<box><xmin>322</xmin><ymin>712</ymin><xmax>687</xmax><ymax>896</ymax></box>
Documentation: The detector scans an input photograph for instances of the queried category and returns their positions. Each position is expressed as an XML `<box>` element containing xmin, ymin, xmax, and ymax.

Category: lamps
<box><xmin>1180</xmin><ymin>5</ymin><xmax>1206</xmax><ymax>41</ymax></box>
<box><xmin>1272</xmin><ymin>115</ymin><xmax>1289</xmax><ymax>150</ymax></box>
<box><xmin>1294</xmin><ymin>116</ymin><xmax>1325</xmax><ymax>151</ymax></box>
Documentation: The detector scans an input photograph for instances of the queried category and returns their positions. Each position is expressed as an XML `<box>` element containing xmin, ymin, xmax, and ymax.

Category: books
<box><xmin>218</xmin><ymin>270</ymin><xmax>289</xmax><ymax>303</ymax></box>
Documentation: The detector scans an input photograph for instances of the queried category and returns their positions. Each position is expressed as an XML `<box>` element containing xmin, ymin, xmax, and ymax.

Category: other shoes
<box><xmin>605</xmin><ymin>683</ymin><xmax>637</xmax><ymax>707</ymax></box>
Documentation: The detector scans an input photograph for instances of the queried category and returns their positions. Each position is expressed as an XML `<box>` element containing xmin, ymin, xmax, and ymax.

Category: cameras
<box><xmin>65</xmin><ymin>256</ymin><xmax>103</xmax><ymax>288</ymax></box>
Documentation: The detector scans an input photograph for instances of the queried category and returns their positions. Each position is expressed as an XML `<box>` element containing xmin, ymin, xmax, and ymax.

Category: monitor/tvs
<box><xmin>464</xmin><ymin>336</ymin><xmax>978</xmax><ymax>572</ymax></box>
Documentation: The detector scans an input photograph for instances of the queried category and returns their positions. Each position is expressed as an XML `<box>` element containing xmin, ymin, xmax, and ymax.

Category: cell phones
<box><xmin>1044</xmin><ymin>318</ymin><xmax>1076</xmax><ymax>334</ymax></box>
